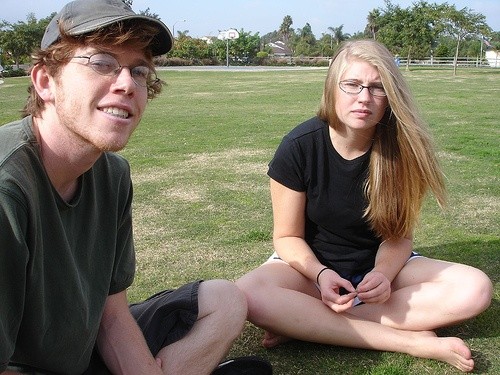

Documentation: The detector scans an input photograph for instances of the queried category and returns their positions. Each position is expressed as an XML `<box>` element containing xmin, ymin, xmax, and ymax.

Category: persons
<box><xmin>0</xmin><ymin>1</ymin><xmax>274</xmax><ymax>375</ymax></box>
<box><xmin>235</xmin><ymin>40</ymin><xmax>492</xmax><ymax>373</ymax></box>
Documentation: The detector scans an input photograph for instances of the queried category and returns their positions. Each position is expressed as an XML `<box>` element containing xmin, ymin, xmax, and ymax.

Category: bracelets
<box><xmin>317</xmin><ymin>268</ymin><xmax>337</xmax><ymax>286</ymax></box>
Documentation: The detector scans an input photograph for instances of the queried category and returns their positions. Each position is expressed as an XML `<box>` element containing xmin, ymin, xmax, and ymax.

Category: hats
<box><xmin>40</xmin><ymin>0</ymin><xmax>174</xmax><ymax>57</ymax></box>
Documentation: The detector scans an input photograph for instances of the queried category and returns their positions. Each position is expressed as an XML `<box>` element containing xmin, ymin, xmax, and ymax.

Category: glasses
<box><xmin>66</xmin><ymin>52</ymin><xmax>160</xmax><ymax>87</ymax></box>
<box><xmin>337</xmin><ymin>80</ymin><xmax>388</xmax><ymax>97</ymax></box>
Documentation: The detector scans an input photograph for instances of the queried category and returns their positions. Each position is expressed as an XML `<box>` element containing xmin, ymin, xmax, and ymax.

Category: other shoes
<box><xmin>211</xmin><ymin>355</ymin><xmax>272</xmax><ymax>375</ymax></box>
